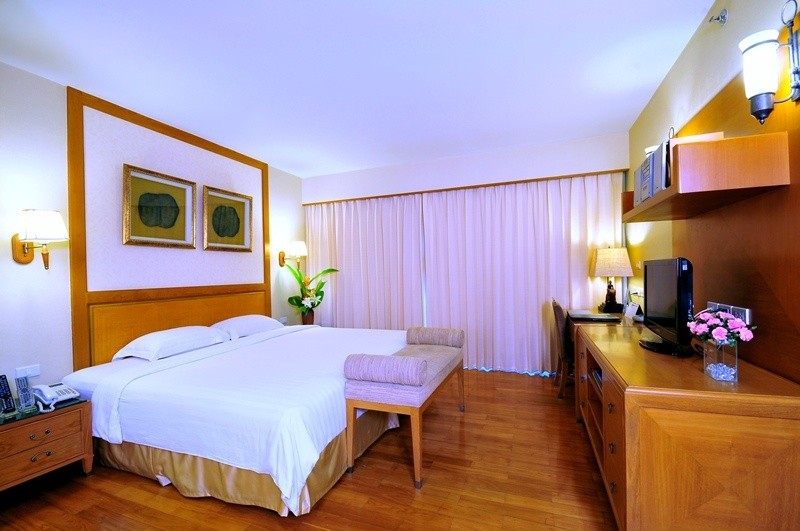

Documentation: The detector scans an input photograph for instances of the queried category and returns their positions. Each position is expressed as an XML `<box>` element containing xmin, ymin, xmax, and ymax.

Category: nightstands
<box><xmin>0</xmin><ymin>398</ymin><xmax>95</xmax><ymax>493</ymax></box>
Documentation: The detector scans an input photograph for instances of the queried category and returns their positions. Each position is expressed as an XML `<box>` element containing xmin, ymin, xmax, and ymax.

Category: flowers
<box><xmin>283</xmin><ymin>262</ymin><xmax>340</xmax><ymax>312</ymax></box>
<box><xmin>687</xmin><ymin>311</ymin><xmax>754</xmax><ymax>345</ymax></box>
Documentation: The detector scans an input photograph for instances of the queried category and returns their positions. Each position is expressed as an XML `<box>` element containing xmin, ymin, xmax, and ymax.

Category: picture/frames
<box><xmin>121</xmin><ymin>162</ymin><xmax>197</xmax><ymax>250</ymax></box>
<box><xmin>202</xmin><ymin>184</ymin><xmax>254</xmax><ymax>254</ymax></box>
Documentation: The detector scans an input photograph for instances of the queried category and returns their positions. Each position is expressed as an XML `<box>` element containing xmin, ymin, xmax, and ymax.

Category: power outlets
<box><xmin>732</xmin><ymin>307</ymin><xmax>750</xmax><ymax>325</ymax></box>
<box><xmin>707</xmin><ymin>301</ymin><xmax>718</xmax><ymax>311</ymax></box>
<box><xmin>718</xmin><ymin>303</ymin><xmax>732</xmax><ymax>314</ymax></box>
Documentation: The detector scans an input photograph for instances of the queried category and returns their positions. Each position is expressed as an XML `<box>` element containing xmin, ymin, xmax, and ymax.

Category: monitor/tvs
<box><xmin>640</xmin><ymin>256</ymin><xmax>694</xmax><ymax>358</ymax></box>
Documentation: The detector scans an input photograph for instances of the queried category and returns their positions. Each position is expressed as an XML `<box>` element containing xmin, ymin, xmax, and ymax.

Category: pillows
<box><xmin>210</xmin><ymin>314</ymin><xmax>285</xmax><ymax>341</ymax></box>
<box><xmin>111</xmin><ymin>325</ymin><xmax>231</xmax><ymax>362</ymax></box>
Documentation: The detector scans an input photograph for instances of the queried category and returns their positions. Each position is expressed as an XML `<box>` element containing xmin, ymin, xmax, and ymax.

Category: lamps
<box><xmin>644</xmin><ymin>144</ymin><xmax>662</xmax><ymax>158</ymax></box>
<box><xmin>737</xmin><ymin>0</ymin><xmax>800</xmax><ymax>125</ymax></box>
<box><xmin>587</xmin><ymin>246</ymin><xmax>633</xmax><ymax>313</ymax></box>
<box><xmin>11</xmin><ymin>208</ymin><xmax>70</xmax><ymax>270</ymax></box>
<box><xmin>278</xmin><ymin>240</ymin><xmax>309</xmax><ymax>267</ymax></box>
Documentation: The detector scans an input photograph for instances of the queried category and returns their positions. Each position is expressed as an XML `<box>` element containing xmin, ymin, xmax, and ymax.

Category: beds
<box><xmin>88</xmin><ymin>281</ymin><xmax>409</xmax><ymax>517</ymax></box>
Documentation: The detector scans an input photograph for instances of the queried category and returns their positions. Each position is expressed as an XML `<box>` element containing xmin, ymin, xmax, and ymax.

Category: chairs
<box><xmin>552</xmin><ymin>299</ymin><xmax>573</xmax><ymax>400</ymax></box>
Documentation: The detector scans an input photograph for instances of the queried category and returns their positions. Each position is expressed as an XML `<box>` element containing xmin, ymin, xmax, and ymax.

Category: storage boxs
<box><xmin>653</xmin><ymin>141</ymin><xmax>663</xmax><ymax>195</ymax></box>
<box><xmin>632</xmin><ymin>166</ymin><xmax>641</xmax><ymax>208</ymax></box>
<box><xmin>641</xmin><ymin>155</ymin><xmax>653</xmax><ymax>204</ymax></box>
<box><xmin>663</xmin><ymin>131</ymin><xmax>735</xmax><ymax>188</ymax></box>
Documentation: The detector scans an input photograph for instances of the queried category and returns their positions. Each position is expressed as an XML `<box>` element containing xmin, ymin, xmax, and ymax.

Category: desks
<box><xmin>565</xmin><ymin>307</ymin><xmax>640</xmax><ymax>326</ymax></box>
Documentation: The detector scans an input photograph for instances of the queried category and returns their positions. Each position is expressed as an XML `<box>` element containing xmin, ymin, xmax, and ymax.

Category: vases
<box><xmin>702</xmin><ymin>341</ymin><xmax>739</xmax><ymax>383</ymax></box>
<box><xmin>301</xmin><ymin>308</ymin><xmax>314</xmax><ymax>324</ymax></box>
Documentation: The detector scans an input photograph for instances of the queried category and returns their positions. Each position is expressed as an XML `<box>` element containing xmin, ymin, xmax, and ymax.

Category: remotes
<box><xmin>16</xmin><ymin>377</ymin><xmax>34</xmax><ymax>409</ymax></box>
<box><xmin>0</xmin><ymin>375</ymin><xmax>15</xmax><ymax>412</ymax></box>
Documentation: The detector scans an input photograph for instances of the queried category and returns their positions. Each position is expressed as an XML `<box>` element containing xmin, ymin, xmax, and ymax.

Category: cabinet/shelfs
<box><xmin>574</xmin><ymin>326</ymin><xmax>800</xmax><ymax>531</ymax></box>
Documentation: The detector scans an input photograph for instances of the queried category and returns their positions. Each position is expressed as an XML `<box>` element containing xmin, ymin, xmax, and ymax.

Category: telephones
<box><xmin>30</xmin><ymin>381</ymin><xmax>80</xmax><ymax>406</ymax></box>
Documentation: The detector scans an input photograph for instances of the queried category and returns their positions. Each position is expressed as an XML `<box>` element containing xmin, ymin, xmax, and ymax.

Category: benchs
<box><xmin>343</xmin><ymin>325</ymin><xmax>466</xmax><ymax>489</ymax></box>
